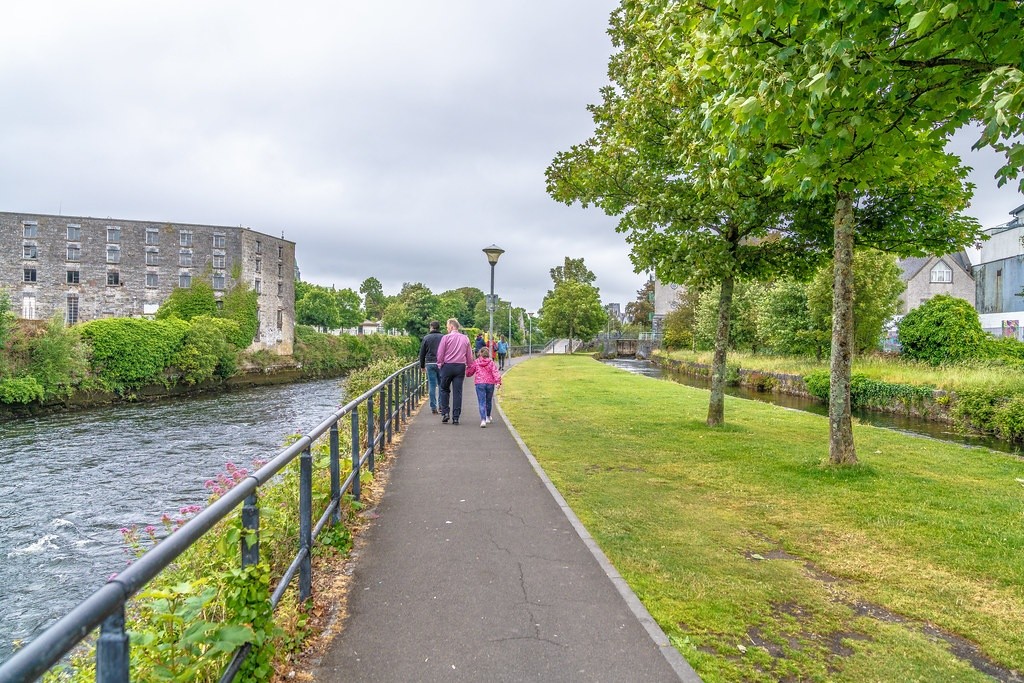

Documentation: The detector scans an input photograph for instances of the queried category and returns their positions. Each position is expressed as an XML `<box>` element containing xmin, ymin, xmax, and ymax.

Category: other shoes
<box><xmin>480</xmin><ymin>422</ymin><xmax>486</xmax><ymax>428</ymax></box>
<box><xmin>453</xmin><ymin>420</ymin><xmax>458</xmax><ymax>425</ymax></box>
<box><xmin>432</xmin><ymin>409</ymin><xmax>437</xmax><ymax>413</ymax></box>
<box><xmin>438</xmin><ymin>409</ymin><xmax>442</xmax><ymax>413</ymax></box>
<box><xmin>442</xmin><ymin>413</ymin><xmax>450</xmax><ymax>422</ymax></box>
<box><xmin>486</xmin><ymin>415</ymin><xmax>492</xmax><ymax>423</ymax></box>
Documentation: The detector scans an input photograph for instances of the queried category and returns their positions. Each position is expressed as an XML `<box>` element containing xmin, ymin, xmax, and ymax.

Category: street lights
<box><xmin>527</xmin><ymin>311</ymin><xmax>535</xmax><ymax>359</ymax></box>
<box><xmin>482</xmin><ymin>242</ymin><xmax>506</xmax><ymax>361</ymax></box>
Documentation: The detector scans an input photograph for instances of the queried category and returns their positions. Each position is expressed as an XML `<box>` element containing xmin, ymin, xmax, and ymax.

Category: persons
<box><xmin>437</xmin><ymin>319</ymin><xmax>474</xmax><ymax>425</ymax></box>
<box><xmin>420</xmin><ymin>321</ymin><xmax>444</xmax><ymax>414</ymax></box>
<box><xmin>466</xmin><ymin>347</ymin><xmax>502</xmax><ymax>427</ymax></box>
<box><xmin>498</xmin><ymin>334</ymin><xmax>508</xmax><ymax>370</ymax></box>
<box><xmin>486</xmin><ymin>337</ymin><xmax>498</xmax><ymax>361</ymax></box>
<box><xmin>474</xmin><ymin>333</ymin><xmax>486</xmax><ymax>359</ymax></box>
